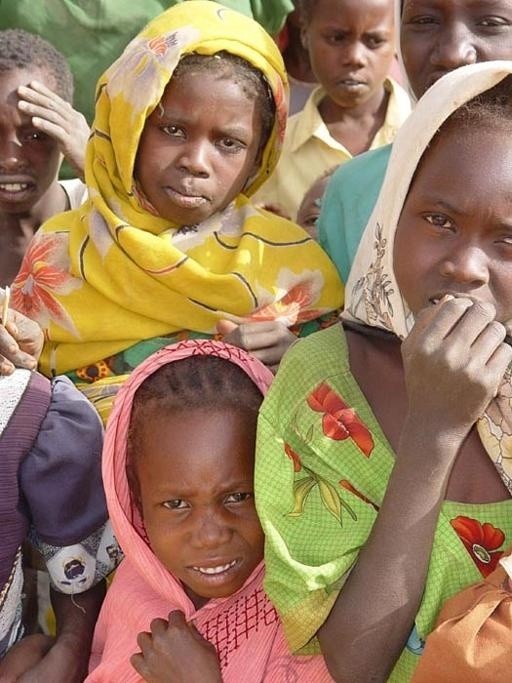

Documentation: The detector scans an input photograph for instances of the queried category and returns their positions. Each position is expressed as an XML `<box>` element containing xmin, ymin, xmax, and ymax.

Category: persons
<box><xmin>278</xmin><ymin>0</ymin><xmax>321</xmax><ymax>116</ymax></box>
<box><xmin>248</xmin><ymin>58</ymin><xmax>512</xmax><ymax>682</ymax></box>
<box><xmin>2</xmin><ymin>362</ymin><xmax>124</xmax><ymax>682</ymax></box>
<box><xmin>261</xmin><ymin>167</ymin><xmax>334</xmax><ymax>238</ymax></box>
<box><xmin>85</xmin><ymin>335</ymin><xmax>336</xmax><ymax>683</ymax></box>
<box><xmin>1</xmin><ymin>26</ymin><xmax>98</xmax><ymax>295</ymax></box>
<box><xmin>316</xmin><ymin>0</ymin><xmax>511</xmax><ymax>290</ymax></box>
<box><xmin>1</xmin><ymin>0</ymin><xmax>355</xmax><ymax>426</ymax></box>
<box><xmin>251</xmin><ymin>0</ymin><xmax>411</xmax><ymax>226</ymax></box>
